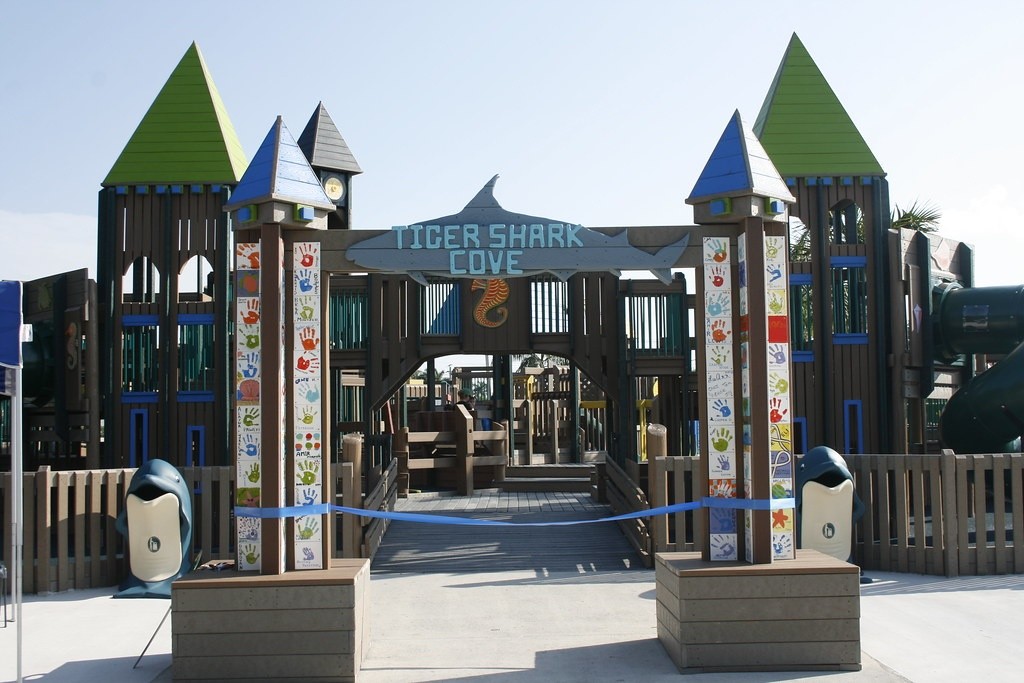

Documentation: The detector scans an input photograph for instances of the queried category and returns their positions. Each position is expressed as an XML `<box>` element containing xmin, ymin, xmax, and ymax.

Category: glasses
<box><xmin>466</xmin><ymin>394</ymin><xmax>471</xmax><ymax>397</ymax></box>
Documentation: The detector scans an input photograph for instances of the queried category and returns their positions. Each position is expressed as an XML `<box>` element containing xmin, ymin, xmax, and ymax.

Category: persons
<box><xmin>451</xmin><ymin>388</ymin><xmax>476</xmax><ymax>412</ymax></box>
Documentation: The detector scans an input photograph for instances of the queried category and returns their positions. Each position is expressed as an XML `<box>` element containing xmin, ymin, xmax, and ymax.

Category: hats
<box><xmin>462</xmin><ymin>388</ymin><xmax>471</xmax><ymax>394</ymax></box>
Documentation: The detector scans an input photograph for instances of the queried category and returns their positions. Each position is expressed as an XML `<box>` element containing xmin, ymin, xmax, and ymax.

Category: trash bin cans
<box><xmin>795</xmin><ymin>446</ymin><xmax>873</xmax><ymax>584</ymax></box>
<box><xmin>113</xmin><ymin>460</ymin><xmax>193</xmax><ymax>599</ymax></box>
